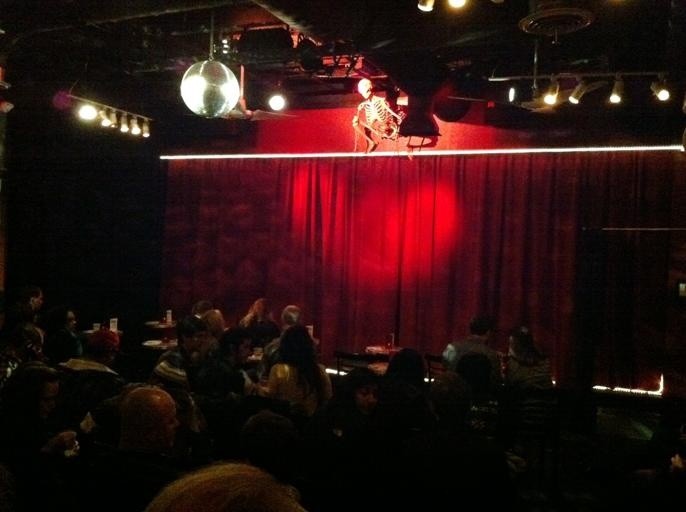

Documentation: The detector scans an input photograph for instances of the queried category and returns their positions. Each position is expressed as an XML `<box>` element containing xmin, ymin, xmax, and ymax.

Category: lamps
<box><xmin>418</xmin><ymin>0</ymin><xmax>435</xmax><ymax>12</ymax></box>
<box><xmin>180</xmin><ymin>0</ymin><xmax>241</xmax><ymax>119</ymax></box>
<box><xmin>487</xmin><ymin>71</ymin><xmax>671</xmax><ymax>105</ymax></box>
<box><xmin>67</xmin><ymin>92</ymin><xmax>153</xmax><ymax>140</ymax></box>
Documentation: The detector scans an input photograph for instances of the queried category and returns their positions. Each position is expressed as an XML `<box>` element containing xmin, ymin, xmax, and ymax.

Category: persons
<box><xmin>1</xmin><ymin>287</ymin><xmax>685</xmax><ymax>512</ymax></box>
